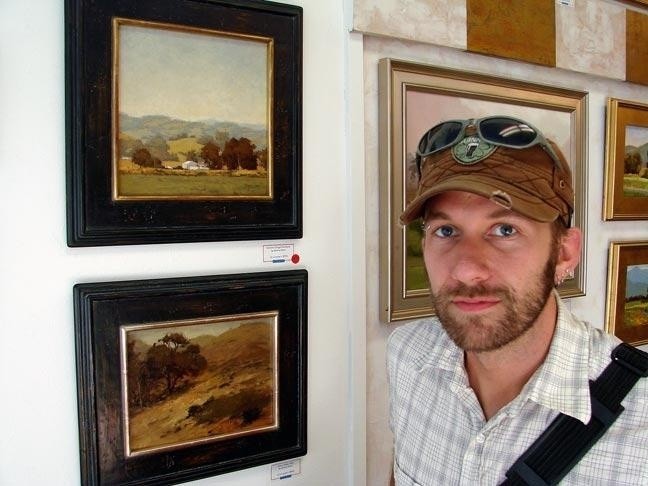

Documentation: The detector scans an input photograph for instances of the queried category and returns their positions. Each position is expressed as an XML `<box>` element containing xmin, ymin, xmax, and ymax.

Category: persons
<box><xmin>383</xmin><ymin>114</ymin><xmax>647</xmax><ymax>485</ymax></box>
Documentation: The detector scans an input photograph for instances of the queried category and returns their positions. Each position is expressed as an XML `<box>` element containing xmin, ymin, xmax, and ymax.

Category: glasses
<box><xmin>415</xmin><ymin>115</ymin><xmax>562</xmax><ymax>179</ymax></box>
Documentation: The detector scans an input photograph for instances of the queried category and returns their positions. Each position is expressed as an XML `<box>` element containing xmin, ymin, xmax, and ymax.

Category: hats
<box><xmin>399</xmin><ymin>125</ymin><xmax>574</xmax><ymax>227</ymax></box>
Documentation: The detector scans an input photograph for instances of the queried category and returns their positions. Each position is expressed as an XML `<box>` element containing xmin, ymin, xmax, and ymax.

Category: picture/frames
<box><xmin>62</xmin><ymin>0</ymin><xmax>306</xmax><ymax>249</ymax></box>
<box><xmin>73</xmin><ymin>267</ymin><xmax>310</xmax><ymax>486</ymax></box>
<box><xmin>376</xmin><ymin>56</ymin><xmax>589</xmax><ymax>328</ymax></box>
<box><xmin>604</xmin><ymin>239</ymin><xmax>647</xmax><ymax>346</ymax></box>
<box><xmin>601</xmin><ymin>94</ymin><xmax>647</xmax><ymax>222</ymax></box>
